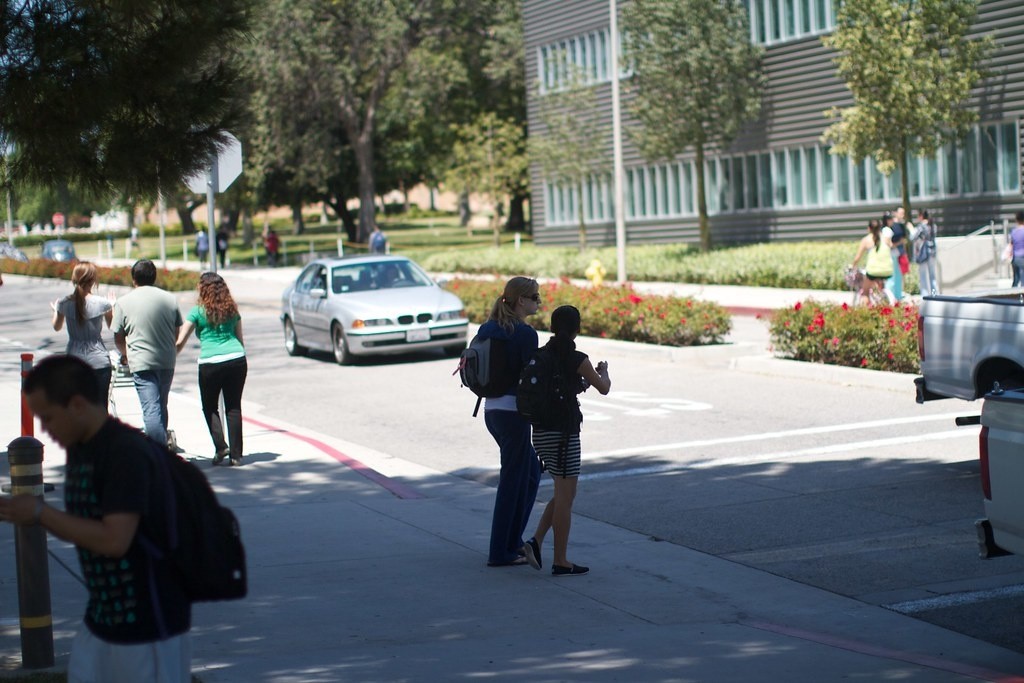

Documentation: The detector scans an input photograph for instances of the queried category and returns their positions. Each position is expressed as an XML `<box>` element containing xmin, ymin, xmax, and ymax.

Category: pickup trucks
<box><xmin>914</xmin><ymin>292</ymin><xmax>1024</xmax><ymax>426</ymax></box>
<box><xmin>972</xmin><ymin>380</ymin><xmax>1024</xmax><ymax>560</ymax></box>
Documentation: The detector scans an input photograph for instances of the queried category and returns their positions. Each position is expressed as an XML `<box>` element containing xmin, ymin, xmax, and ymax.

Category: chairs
<box><xmin>334</xmin><ymin>276</ymin><xmax>354</xmax><ymax>292</ymax></box>
<box><xmin>360</xmin><ymin>269</ymin><xmax>372</xmax><ymax>283</ymax></box>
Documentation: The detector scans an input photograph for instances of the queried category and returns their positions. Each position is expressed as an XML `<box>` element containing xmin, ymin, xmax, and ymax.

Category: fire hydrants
<box><xmin>583</xmin><ymin>260</ymin><xmax>606</xmax><ymax>286</ymax></box>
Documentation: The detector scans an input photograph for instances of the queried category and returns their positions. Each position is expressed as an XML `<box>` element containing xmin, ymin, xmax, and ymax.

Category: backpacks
<box><xmin>145</xmin><ymin>436</ymin><xmax>246</xmax><ymax>603</ymax></box>
<box><xmin>516</xmin><ymin>358</ymin><xmax>582</xmax><ymax>431</ymax></box>
<box><xmin>458</xmin><ymin>332</ymin><xmax>515</xmax><ymax>398</ymax></box>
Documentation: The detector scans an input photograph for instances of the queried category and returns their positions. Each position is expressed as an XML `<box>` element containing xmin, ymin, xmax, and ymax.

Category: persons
<box><xmin>459</xmin><ymin>193</ymin><xmax>469</xmax><ymax>226</ymax></box>
<box><xmin>111</xmin><ymin>260</ymin><xmax>183</xmax><ymax>449</ymax></box>
<box><xmin>525</xmin><ymin>305</ymin><xmax>612</xmax><ymax>576</ymax></box>
<box><xmin>851</xmin><ymin>206</ymin><xmax>938</xmax><ymax>306</ymax></box>
<box><xmin>195</xmin><ymin>225</ymin><xmax>230</xmax><ymax>270</ymax></box>
<box><xmin>1008</xmin><ymin>210</ymin><xmax>1024</xmax><ymax>287</ymax></box>
<box><xmin>263</xmin><ymin>225</ymin><xmax>280</xmax><ymax>264</ymax></box>
<box><xmin>131</xmin><ymin>224</ymin><xmax>141</xmax><ymax>251</ymax></box>
<box><xmin>476</xmin><ymin>278</ymin><xmax>541</xmax><ymax>567</ymax></box>
<box><xmin>368</xmin><ymin>223</ymin><xmax>390</xmax><ymax>255</ymax></box>
<box><xmin>107</xmin><ymin>231</ymin><xmax>113</xmax><ymax>249</ymax></box>
<box><xmin>175</xmin><ymin>272</ymin><xmax>248</xmax><ymax>465</ymax></box>
<box><xmin>50</xmin><ymin>264</ymin><xmax>113</xmax><ymax>414</ymax></box>
<box><xmin>0</xmin><ymin>353</ymin><xmax>191</xmax><ymax>683</ymax></box>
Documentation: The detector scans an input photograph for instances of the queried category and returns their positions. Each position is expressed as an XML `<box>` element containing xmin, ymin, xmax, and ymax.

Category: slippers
<box><xmin>487</xmin><ymin>553</ymin><xmax>529</xmax><ymax>567</ymax></box>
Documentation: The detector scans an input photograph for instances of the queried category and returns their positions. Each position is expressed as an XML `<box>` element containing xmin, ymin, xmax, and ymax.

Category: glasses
<box><xmin>523</xmin><ymin>293</ymin><xmax>539</xmax><ymax>302</ymax></box>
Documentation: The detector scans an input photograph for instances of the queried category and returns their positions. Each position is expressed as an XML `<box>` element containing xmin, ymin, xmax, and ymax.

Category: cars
<box><xmin>280</xmin><ymin>253</ymin><xmax>468</xmax><ymax>367</ymax></box>
<box><xmin>41</xmin><ymin>240</ymin><xmax>75</xmax><ymax>262</ymax></box>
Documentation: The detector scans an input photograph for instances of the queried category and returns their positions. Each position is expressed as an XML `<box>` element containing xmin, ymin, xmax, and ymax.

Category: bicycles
<box><xmin>845</xmin><ymin>264</ymin><xmax>896</xmax><ymax>306</ymax></box>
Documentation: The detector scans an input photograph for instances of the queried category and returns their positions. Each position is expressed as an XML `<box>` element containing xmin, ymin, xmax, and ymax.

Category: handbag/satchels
<box><xmin>909</xmin><ymin>237</ymin><xmax>928</xmax><ymax>264</ymax></box>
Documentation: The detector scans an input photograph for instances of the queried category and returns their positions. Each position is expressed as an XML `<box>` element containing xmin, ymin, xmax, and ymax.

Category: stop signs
<box><xmin>52</xmin><ymin>212</ymin><xmax>64</xmax><ymax>225</ymax></box>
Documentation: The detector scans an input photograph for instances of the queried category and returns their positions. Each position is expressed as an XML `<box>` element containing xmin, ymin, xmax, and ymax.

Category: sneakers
<box><xmin>551</xmin><ymin>562</ymin><xmax>589</xmax><ymax>576</ymax></box>
<box><xmin>525</xmin><ymin>537</ymin><xmax>543</xmax><ymax>570</ymax></box>
<box><xmin>212</xmin><ymin>448</ymin><xmax>230</xmax><ymax>464</ymax></box>
<box><xmin>230</xmin><ymin>457</ymin><xmax>243</xmax><ymax>466</ymax></box>
